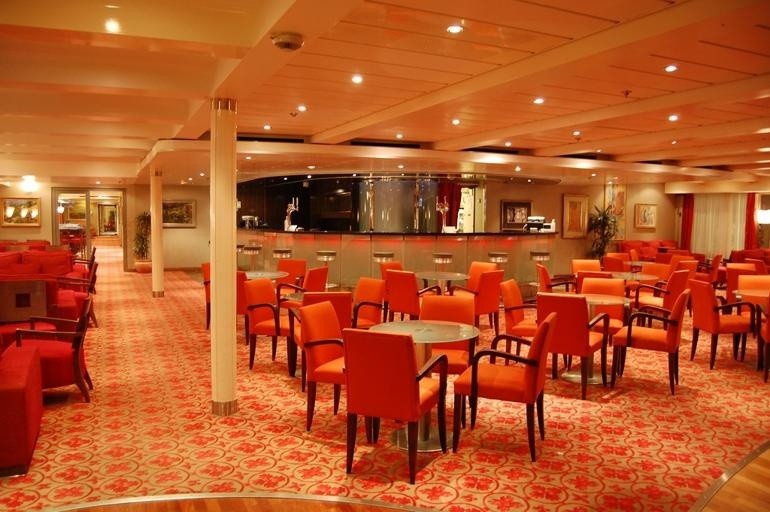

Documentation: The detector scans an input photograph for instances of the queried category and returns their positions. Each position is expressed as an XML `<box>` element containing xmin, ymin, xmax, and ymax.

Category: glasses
<box><xmin>756</xmin><ymin>208</ymin><xmax>770</xmax><ymax>244</ymax></box>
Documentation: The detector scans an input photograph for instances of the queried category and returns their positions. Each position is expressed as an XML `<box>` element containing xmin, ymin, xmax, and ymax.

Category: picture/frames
<box><xmin>634</xmin><ymin>204</ymin><xmax>657</xmax><ymax>230</ymax></box>
<box><xmin>68</xmin><ymin>205</ymin><xmax>86</xmax><ymax>221</ymax></box>
<box><xmin>499</xmin><ymin>199</ymin><xmax>534</xmax><ymax>232</ymax></box>
<box><xmin>603</xmin><ymin>181</ymin><xmax>627</xmax><ymax>243</ymax></box>
<box><xmin>159</xmin><ymin>199</ymin><xmax>196</xmax><ymax>228</ymax></box>
<box><xmin>563</xmin><ymin>192</ymin><xmax>590</xmax><ymax>237</ymax></box>
<box><xmin>1</xmin><ymin>197</ymin><xmax>41</xmax><ymax>227</ymax></box>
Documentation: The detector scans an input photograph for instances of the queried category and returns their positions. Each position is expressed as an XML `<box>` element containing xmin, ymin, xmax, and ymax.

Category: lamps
<box><xmin>1</xmin><ymin>224</ymin><xmax>100</xmax><ymax>476</ymax></box>
<box><xmin>756</xmin><ymin>208</ymin><xmax>770</xmax><ymax>244</ymax></box>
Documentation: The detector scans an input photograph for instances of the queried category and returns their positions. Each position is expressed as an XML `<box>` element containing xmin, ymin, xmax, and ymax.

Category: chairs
<box><xmin>203</xmin><ymin>259</ymin><xmax>504</xmax><ymax>392</ymax></box>
<box><xmin>342</xmin><ymin>328</ymin><xmax>448</xmax><ymax>483</ymax></box>
<box><xmin>453</xmin><ymin>311</ymin><xmax>556</xmax><ymax>462</ymax></box>
<box><xmin>420</xmin><ymin>294</ymin><xmax>476</xmax><ymax>428</ymax></box>
<box><xmin>1</xmin><ymin>224</ymin><xmax>100</xmax><ymax>476</ymax></box>
<box><xmin>299</xmin><ymin>301</ymin><xmax>348</xmax><ymax>432</ymax></box>
<box><xmin>500</xmin><ymin>239</ymin><xmax>769</xmax><ymax>400</ymax></box>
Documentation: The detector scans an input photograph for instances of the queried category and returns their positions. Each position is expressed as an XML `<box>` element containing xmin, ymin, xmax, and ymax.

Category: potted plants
<box><xmin>1</xmin><ymin>197</ymin><xmax>41</xmax><ymax>227</ymax></box>
<box><xmin>68</xmin><ymin>205</ymin><xmax>86</xmax><ymax>221</ymax></box>
<box><xmin>563</xmin><ymin>192</ymin><xmax>590</xmax><ymax>237</ymax></box>
<box><xmin>132</xmin><ymin>211</ymin><xmax>153</xmax><ymax>273</ymax></box>
<box><xmin>603</xmin><ymin>181</ymin><xmax>627</xmax><ymax>243</ymax></box>
<box><xmin>634</xmin><ymin>204</ymin><xmax>657</xmax><ymax>230</ymax></box>
<box><xmin>499</xmin><ymin>199</ymin><xmax>534</xmax><ymax>232</ymax></box>
<box><xmin>159</xmin><ymin>199</ymin><xmax>196</xmax><ymax>228</ymax></box>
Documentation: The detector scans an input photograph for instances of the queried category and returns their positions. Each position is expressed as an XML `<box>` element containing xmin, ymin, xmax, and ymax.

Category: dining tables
<box><xmin>132</xmin><ymin>211</ymin><xmax>153</xmax><ymax>273</ymax></box>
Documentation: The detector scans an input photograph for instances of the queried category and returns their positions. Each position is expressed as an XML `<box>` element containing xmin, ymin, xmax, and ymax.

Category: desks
<box><xmin>370</xmin><ymin>320</ymin><xmax>481</xmax><ymax>453</ymax></box>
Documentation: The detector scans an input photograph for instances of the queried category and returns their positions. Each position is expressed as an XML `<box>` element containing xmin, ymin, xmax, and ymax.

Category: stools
<box><xmin>236</xmin><ymin>245</ymin><xmax>244</xmax><ymax>264</ymax></box>
<box><xmin>273</xmin><ymin>248</ymin><xmax>292</xmax><ymax>258</ymax></box>
<box><xmin>432</xmin><ymin>253</ymin><xmax>452</xmax><ymax>272</ymax></box>
<box><xmin>316</xmin><ymin>251</ymin><xmax>336</xmax><ymax>266</ymax></box>
<box><xmin>243</xmin><ymin>247</ymin><xmax>261</xmax><ymax>271</ymax></box>
<box><xmin>489</xmin><ymin>252</ymin><xmax>511</xmax><ymax>308</ymax></box>
<box><xmin>373</xmin><ymin>252</ymin><xmax>394</xmax><ymax>279</ymax></box>
